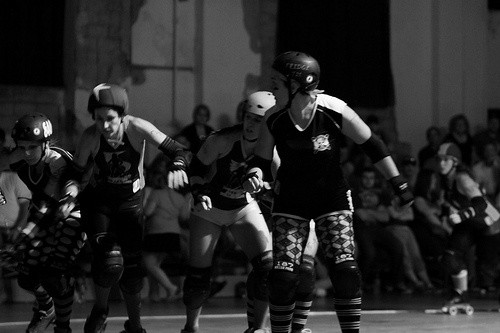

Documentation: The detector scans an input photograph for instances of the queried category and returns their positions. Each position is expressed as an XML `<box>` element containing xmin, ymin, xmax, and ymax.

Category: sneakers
<box><xmin>83</xmin><ymin>305</ymin><xmax>109</xmax><ymax>333</ymax></box>
<box><xmin>25</xmin><ymin>300</ymin><xmax>55</xmax><ymax>333</ymax></box>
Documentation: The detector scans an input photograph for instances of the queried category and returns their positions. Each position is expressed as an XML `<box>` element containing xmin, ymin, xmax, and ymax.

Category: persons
<box><xmin>59</xmin><ymin>84</ymin><xmax>192</xmax><ymax>333</ymax></box>
<box><xmin>313</xmin><ymin>108</ymin><xmax>500</xmax><ymax>314</ymax></box>
<box><xmin>1</xmin><ymin>113</ymin><xmax>88</xmax><ymax>333</ymax></box>
<box><xmin>241</xmin><ymin>51</ymin><xmax>415</xmax><ymax>333</ymax></box>
<box><xmin>137</xmin><ymin>98</ymin><xmax>274</xmax><ymax>305</ymax></box>
<box><xmin>181</xmin><ymin>91</ymin><xmax>280</xmax><ymax>333</ymax></box>
<box><xmin>0</xmin><ymin>129</ymin><xmax>31</xmax><ymax>305</ymax></box>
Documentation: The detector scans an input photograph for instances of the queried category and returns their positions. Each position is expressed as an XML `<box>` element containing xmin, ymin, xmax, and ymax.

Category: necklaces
<box><xmin>243</xmin><ymin>136</ymin><xmax>258</xmax><ymax>142</ymax></box>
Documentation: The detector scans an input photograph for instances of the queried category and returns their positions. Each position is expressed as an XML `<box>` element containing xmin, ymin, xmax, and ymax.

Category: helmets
<box><xmin>245</xmin><ymin>91</ymin><xmax>276</xmax><ymax>115</ymax></box>
<box><xmin>437</xmin><ymin>142</ymin><xmax>461</xmax><ymax>163</ymax></box>
<box><xmin>87</xmin><ymin>82</ymin><xmax>129</xmax><ymax>116</ymax></box>
<box><xmin>10</xmin><ymin>112</ymin><xmax>53</xmax><ymax>141</ymax></box>
<box><xmin>272</xmin><ymin>51</ymin><xmax>320</xmax><ymax>92</ymax></box>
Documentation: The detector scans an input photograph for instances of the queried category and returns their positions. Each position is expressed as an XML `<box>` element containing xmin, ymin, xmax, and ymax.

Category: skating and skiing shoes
<box><xmin>442</xmin><ymin>290</ymin><xmax>474</xmax><ymax>316</ymax></box>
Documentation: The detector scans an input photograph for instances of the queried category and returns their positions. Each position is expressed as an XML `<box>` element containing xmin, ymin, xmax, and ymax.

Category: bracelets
<box><xmin>14</xmin><ymin>225</ymin><xmax>22</xmax><ymax>231</ymax></box>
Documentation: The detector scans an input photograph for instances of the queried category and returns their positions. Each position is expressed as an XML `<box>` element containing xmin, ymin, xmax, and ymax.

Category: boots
<box><xmin>413</xmin><ymin>256</ymin><xmax>435</xmax><ymax>288</ymax></box>
<box><xmin>405</xmin><ymin>263</ymin><xmax>423</xmax><ymax>288</ymax></box>
